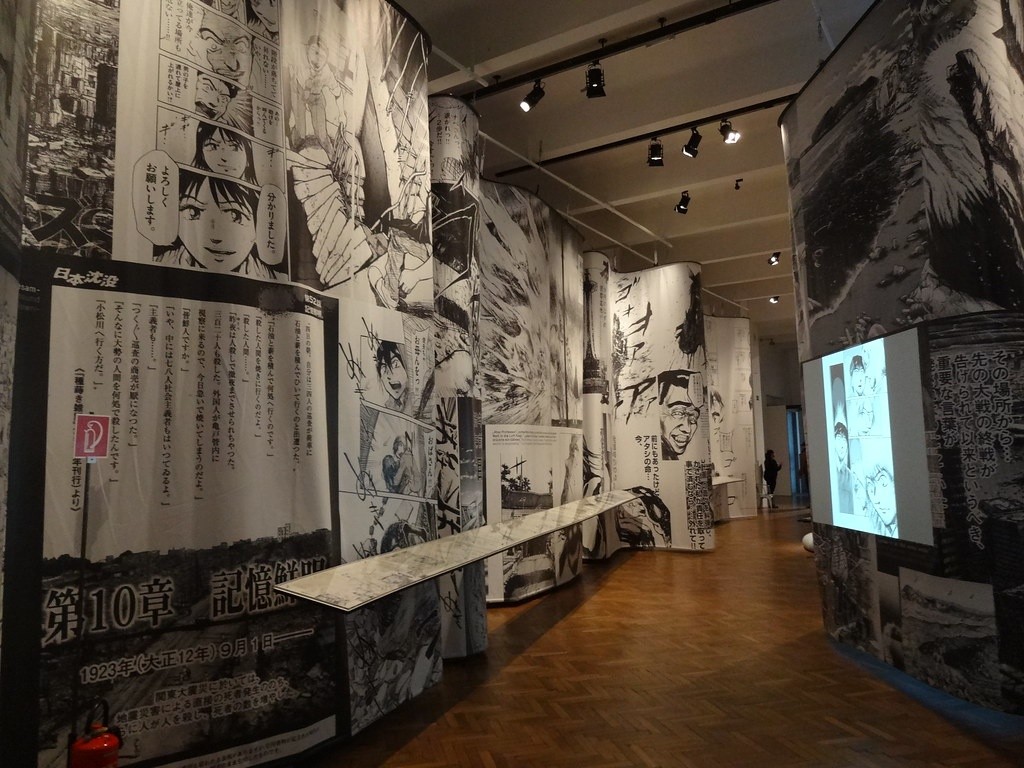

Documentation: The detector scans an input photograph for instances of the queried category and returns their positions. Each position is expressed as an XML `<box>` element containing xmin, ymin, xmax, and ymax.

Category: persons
<box><xmin>796</xmin><ymin>442</ymin><xmax>811</xmax><ymax>509</ymax></box>
<box><xmin>761</xmin><ymin>449</ymin><xmax>784</xmax><ymax>509</ymax></box>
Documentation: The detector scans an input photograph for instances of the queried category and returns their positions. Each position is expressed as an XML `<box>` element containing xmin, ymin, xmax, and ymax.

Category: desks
<box><xmin>710</xmin><ymin>476</ymin><xmax>746</xmax><ymax>490</ymax></box>
<box><xmin>272</xmin><ymin>489</ymin><xmax>647</xmax><ymax>753</ymax></box>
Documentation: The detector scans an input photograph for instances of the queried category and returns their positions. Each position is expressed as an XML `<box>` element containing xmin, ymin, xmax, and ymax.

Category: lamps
<box><xmin>717</xmin><ymin>117</ymin><xmax>744</xmax><ymax>146</ymax></box>
<box><xmin>646</xmin><ymin>137</ymin><xmax>665</xmax><ymax>167</ymax></box>
<box><xmin>768</xmin><ymin>252</ymin><xmax>783</xmax><ymax>267</ymax></box>
<box><xmin>582</xmin><ymin>60</ymin><xmax>607</xmax><ymax>99</ymax></box>
<box><xmin>519</xmin><ymin>79</ymin><xmax>546</xmax><ymax>112</ymax></box>
<box><xmin>680</xmin><ymin>128</ymin><xmax>703</xmax><ymax>158</ymax></box>
<box><xmin>673</xmin><ymin>190</ymin><xmax>691</xmax><ymax>215</ymax></box>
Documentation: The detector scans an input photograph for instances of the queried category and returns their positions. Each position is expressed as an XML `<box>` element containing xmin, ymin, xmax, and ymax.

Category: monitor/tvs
<box><xmin>799</xmin><ymin>321</ymin><xmax>937</xmax><ymax>550</ymax></box>
